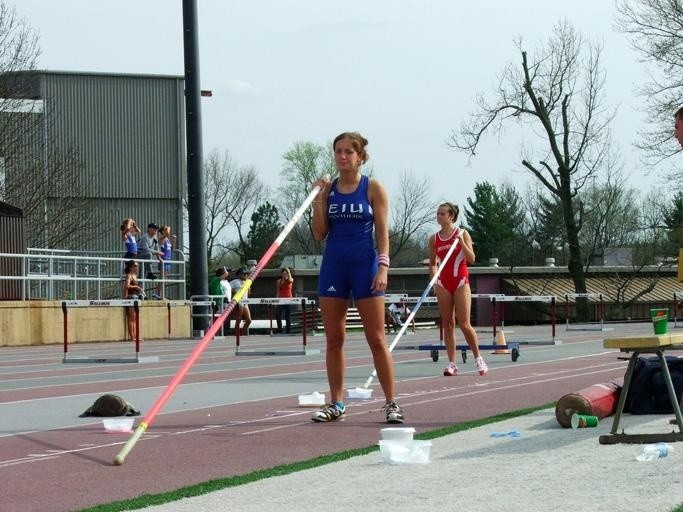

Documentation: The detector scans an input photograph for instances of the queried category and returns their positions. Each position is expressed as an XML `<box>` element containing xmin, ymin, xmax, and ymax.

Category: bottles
<box><xmin>634</xmin><ymin>442</ymin><xmax>675</xmax><ymax>460</ymax></box>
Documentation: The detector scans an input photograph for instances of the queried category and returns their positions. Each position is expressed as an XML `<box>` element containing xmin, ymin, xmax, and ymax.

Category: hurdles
<box><xmin>236</xmin><ymin>297</ymin><xmax>308</xmax><ymax>356</ymax></box>
<box><xmin>62</xmin><ymin>299</ymin><xmax>159</xmax><ymax>363</ymax></box>
<box><xmin>674</xmin><ymin>291</ymin><xmax>683</xmax><ymax>329</ymax></box>
<box><xmin>565</xmin><ymin>292</ymin><xmax>603</xmax><ymax>332</ymax></box>
<box><xmin>490</xmin><ymin>295</ymin><xmax>556</xmax><ymax>344</ymax></box>
<box><xmin>385</xmin><ymin>296</ymin><xmax>439</xmax><ymax>335</ymax></box>
<box><xmin>167</xmin><ymin>301</ymin><xmax>217</xmax><ymax>340</ymax></box>
<box><xmin>440</xmin><ymin>293</ymin><xmax>505</xmax><ymax>348</ymax></box>
<box><xmin>268</xmin><ymin>300</ymin><xmax>316</xmax><ymax>336</ymax></box>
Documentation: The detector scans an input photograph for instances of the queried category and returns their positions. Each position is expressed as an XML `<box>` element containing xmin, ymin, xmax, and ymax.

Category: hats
<box><xmin>148</xmin><ymin>223</ymin><xmax>159</xmax><ymax>229</ymax></box>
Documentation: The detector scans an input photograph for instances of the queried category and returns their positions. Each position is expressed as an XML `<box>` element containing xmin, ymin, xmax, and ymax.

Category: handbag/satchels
<box><xmin>615</xmin><ymin>355</ymin><xmax>681</xmax><ymax>415</ymax></box>
<box><xmin>79</xmin><ymin>394</ymin><xmax>140</xmax><ymax>417</ymax></box>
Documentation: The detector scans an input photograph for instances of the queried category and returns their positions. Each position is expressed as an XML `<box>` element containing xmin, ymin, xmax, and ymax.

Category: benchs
<box><xmin>599</xmin><ymin>333</ymin><xmax>683</xmax><ymax>444</ymax></box>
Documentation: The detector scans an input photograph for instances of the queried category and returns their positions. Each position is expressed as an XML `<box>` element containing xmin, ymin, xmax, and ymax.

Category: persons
<box><xmin>124</xmin><ymin>260</ymin><xmax>144</xmax><ymax>343</ymax></box>
<box><xmin>229</xmin><ymin>269</ymin><xmax>252</xmax><ymax>335</ymax></box>
<box><xmin>427</xmin><ymin>202</ymin><xmax>491</xmax><ymax>375</ymax></box>
<box><xmin>219</xmin><ymin>268</ymin><xmax>234</xmax><ymax>336</ymax></box>
<box><xmin>207</xmin><ymin>265</ymin><xmax>228</xmax><ymax>335</ymax></box>
<box><xmin>120</xmin><ymin>219</ymin><xmax>141</xmax><ymax>299</ymax></box>
<box><xmin>387</xmin><ymin>303</ymin><xmax>417</xmax><ymax>332</ymax></box>
<box><xmin>136</xmin><ymin>223</ymin><xmax>165</xmax><ymax>280</ymax></box>
<box><xmin>312</xmin><ymin>132</ymin><xmax>405</xmax><ymax>424</ymax></box>
<box><xmin>154</xmin><ymin>226</ymin><xmax>177</xmax><ymax>300</ymax></box>
<box><xmin>276</xmin><ymin>267</ymin><xmax>293</xmax><ymax>335</ymax></box>
<box><xmin>672</xmin><ymin>105</ymin><xmax>683</xmax><ymax>146</ymax></box>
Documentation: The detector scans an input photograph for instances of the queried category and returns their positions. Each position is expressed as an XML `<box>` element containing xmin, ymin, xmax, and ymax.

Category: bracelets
<box><xmin>313</xmin><ymin>200</ymin><xmax>325</xmax><ymax>204</ymax></box>
<box><xmin>377</xmin><ymin>253</ymin><xmax>390</xmax><ymax>268</ymax></box>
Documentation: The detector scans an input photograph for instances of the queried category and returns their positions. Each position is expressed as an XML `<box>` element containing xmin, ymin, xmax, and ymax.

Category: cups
<box><xmin>651</xmin><ymin>308</ymin><xmax>668</xmax><ymax>336</ymax></box>
<box><xmin>571</xmin><ymin>413</ymin><xmax>599</xmax><ymax>429</ymax></box>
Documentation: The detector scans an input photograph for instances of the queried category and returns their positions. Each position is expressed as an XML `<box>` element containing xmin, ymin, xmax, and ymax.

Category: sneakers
<box><xmin>444</xmin><ymin>362</ymin><xmax>459</xmax><ymax>376</ymax></box>
<box><xmin>146</xmin><ymin>272</ymin><xmax>157</xmax><ymax>279</ymax></box>
<box><xmin>475</xmin><ymin>356</ymin><xmax>488</xmax><ymax>376</ymax></box>
<box><xmin>312</xmin><ymin>405</ymin><xmax>346</xmax><ymax>422</ymax></box>
<box><xmin>386</xmin><ymin>403</ymin><xmax>404</xmax><ymax>423</ymax></box>
<box><xmin>152</xmin><ymin>294</ymin><xmax>160</xmax><ymax>299</ymax></box>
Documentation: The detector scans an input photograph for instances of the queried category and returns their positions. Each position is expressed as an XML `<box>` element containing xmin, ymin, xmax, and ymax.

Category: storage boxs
<box><xmin>298</xmin><ymin>394</ymin><xmax>325</xmax><ymax>408</ymax></box>
<box><xmin>346</xmin><ymin>388</ymin><xmax>373</xmax><ymax>400</ymax></box>
<box><xmin>377</xmin><ymin>439</ymin><xmax>432</xmax><ymax>465</ymax></box>
<box><xmin>379</xmin><ymin>426</ymin><xmax>416</xmax><ymax>440</ymax></box>
